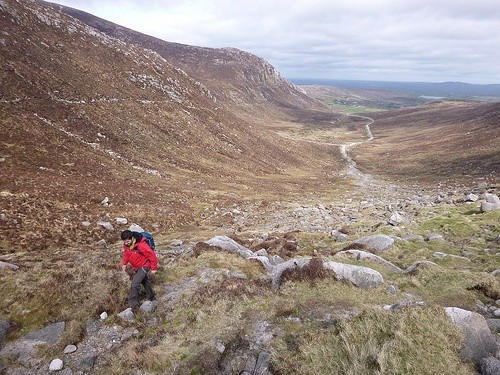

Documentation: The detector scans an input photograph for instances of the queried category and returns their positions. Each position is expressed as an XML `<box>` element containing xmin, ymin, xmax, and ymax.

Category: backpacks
<box><xmin>138</xmin><ymin>231</ymin><xmax>155</xmax><ymax>251</ymax></box>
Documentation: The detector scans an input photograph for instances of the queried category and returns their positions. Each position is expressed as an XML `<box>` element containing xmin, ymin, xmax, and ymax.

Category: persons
<box><xmin>121</xmin><ymin>230</ymin><xmax>158</xmax><ymax>314</ymax></box>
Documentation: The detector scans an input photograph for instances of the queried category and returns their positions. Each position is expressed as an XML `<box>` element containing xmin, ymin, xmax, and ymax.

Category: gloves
<box><xmin>146</xmin><ymin>269</ymin><xmax>156</xmax><ymax>279</ymax></box>
<box><xmin>123</xmin><ymin>265</ymin><xmax>129</xmax><ymax>275</ymax></box>
